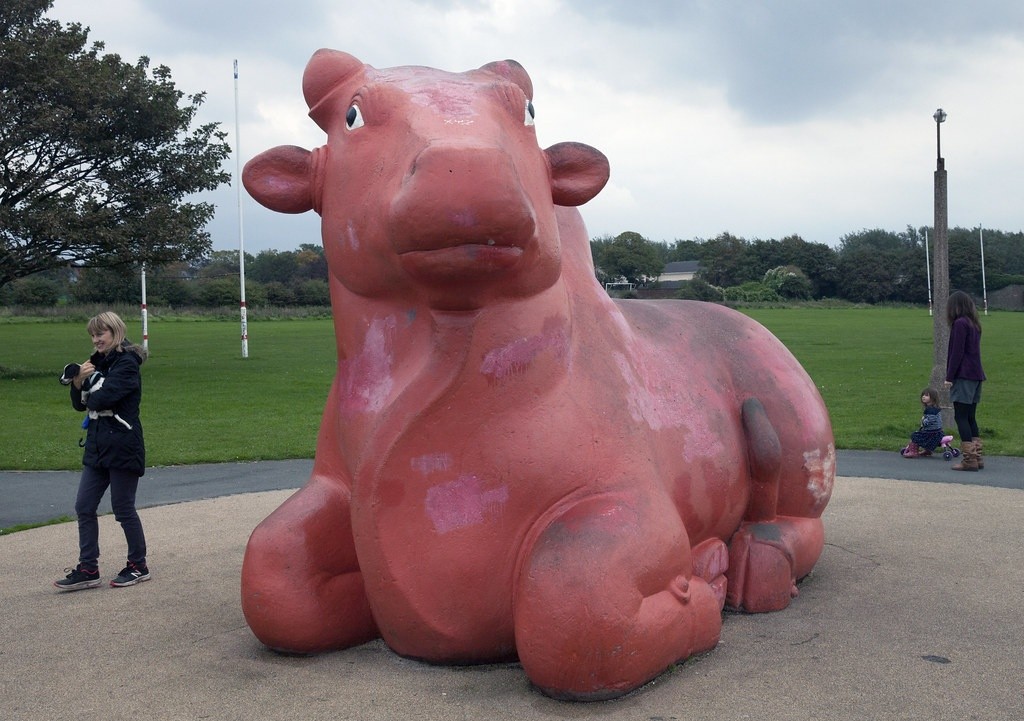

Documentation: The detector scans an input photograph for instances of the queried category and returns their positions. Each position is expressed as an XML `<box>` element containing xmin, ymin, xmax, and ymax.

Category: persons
<box><xmin>54</xmin><ymin>312</ymin><xmax>151</xmax><ymax>592</ymax></box>
<box><xmin>909</xmin><ymin>387</ymin><xmax>944</xmax><ymax>454</ymax></box>
<box><xmin>944</xmin><ymin>291</ymin><xmax>988</xmax><ymax>471</ymax></box>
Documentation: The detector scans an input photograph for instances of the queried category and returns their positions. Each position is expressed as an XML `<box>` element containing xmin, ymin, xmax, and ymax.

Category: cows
<box><xmin>242</xmin><ymin>47</ymin><xmax>837</xmax><ymax>703</ymax></box>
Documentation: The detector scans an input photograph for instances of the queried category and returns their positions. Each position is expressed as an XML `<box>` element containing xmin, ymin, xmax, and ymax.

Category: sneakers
<box><xmin>54</xmin><ymin>564</ymin><xmax>102</xmax><ymax>588</ymax></box>
<box><xmin>110</xmin><ymin>558</ymin><xmax>150</xmax><ymax>586</ymax></box>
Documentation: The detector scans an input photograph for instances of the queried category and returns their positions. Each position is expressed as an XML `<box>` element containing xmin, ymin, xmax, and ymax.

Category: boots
<box><xmin>973</xmin><ymin>437</ymin><xmax>984</xmax><ymax>468</ymax></box>
<box><xmin>951</xmin><ymin>442</ymin><xmax>978</xmax><ymax>471</ymax></box>
<box><xmin>903</xmin><ymin>443</ymin><xmax>919</xmax><ymax>457</ymax></box>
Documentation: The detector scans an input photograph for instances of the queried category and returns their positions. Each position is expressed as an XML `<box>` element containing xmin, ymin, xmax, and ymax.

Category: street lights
<box><xmin>932</xmin><ymin>108</ymin><xmax>953</xmax><ymax>409</ymax></box>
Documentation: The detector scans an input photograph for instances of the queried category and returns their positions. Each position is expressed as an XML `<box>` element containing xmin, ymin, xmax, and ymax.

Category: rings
<box><xmin>949</xmin><ymin>385</ymin><xmax>950</xmax><ymax>386</ymax></box>
